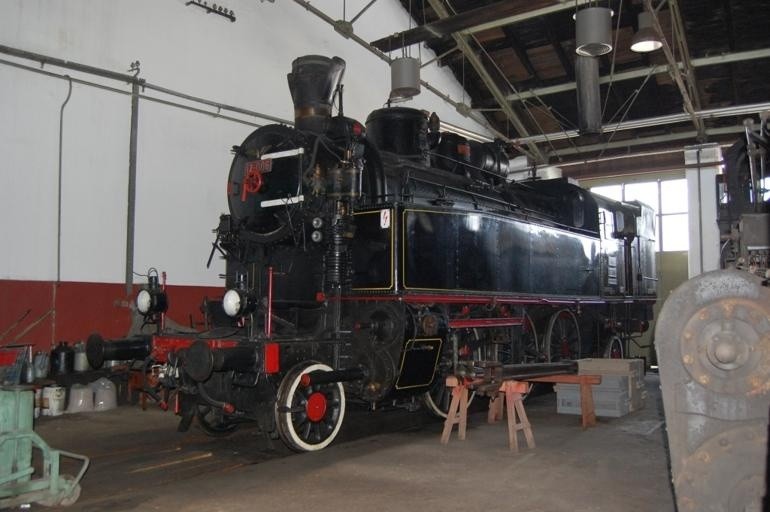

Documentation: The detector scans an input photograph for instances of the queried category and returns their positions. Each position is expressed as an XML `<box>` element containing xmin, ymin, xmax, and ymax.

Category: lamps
<box><xmin>574</xmin><ymin>1</ymin><xmax>664</xmax><ymax>58</ymax></box>
<box><xmin>387</xmin><ymin>32</ymin><xmax>422</xmax><ymax>97</ymax></box>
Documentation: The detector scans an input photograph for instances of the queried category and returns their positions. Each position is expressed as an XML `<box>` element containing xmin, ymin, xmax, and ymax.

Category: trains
<box><xmin>85</xmin><ymin>55</ymin><xmax>659</xmax><ymax>452</ymax></box>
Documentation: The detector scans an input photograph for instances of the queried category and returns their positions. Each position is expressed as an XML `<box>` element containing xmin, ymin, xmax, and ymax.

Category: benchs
<box><xmin>441</xmin><ymin>372</ymin><xmax>601</xmax><ymax>451</ymax></box>
